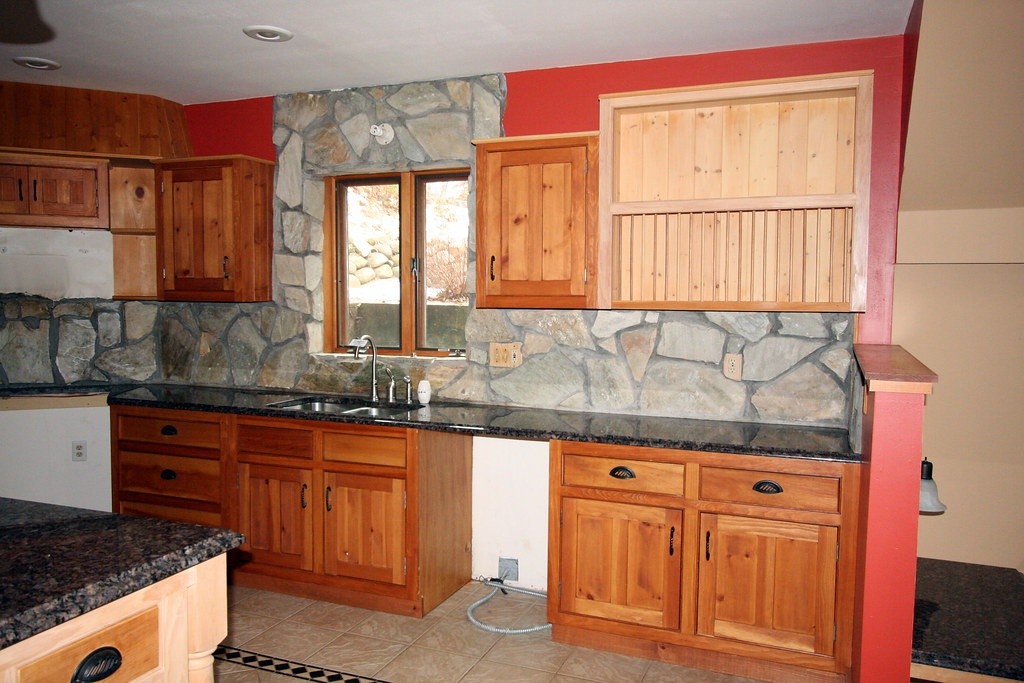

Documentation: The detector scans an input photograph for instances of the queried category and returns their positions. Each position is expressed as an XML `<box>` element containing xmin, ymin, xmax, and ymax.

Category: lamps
<box><xmin>918</xmin><ymin>457</ymin><xmax>947</xmax><ymax>511</ymax></box>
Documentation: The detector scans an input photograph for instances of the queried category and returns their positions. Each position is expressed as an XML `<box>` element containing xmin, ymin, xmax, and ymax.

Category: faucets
<box><xmin>354</xmin><ymin>335</ymin><xmax>381</xmax><ymax>403</ymax></box>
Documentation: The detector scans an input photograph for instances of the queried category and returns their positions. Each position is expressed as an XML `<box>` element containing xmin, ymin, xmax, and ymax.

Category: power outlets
<box><xmin>72</xmin><ymin>440</ymin><xmax>87</xmax><ymax>461</ymax></box>
<box><xmin>723</xmin><ymin>352</ymin><xmax>742</xmax><ymax>381</ymax></box>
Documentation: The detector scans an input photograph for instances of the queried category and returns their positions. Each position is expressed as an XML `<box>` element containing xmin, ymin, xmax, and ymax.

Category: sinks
<box><xmin>343</xmin><ymin>406</ymin><xmax>418</xmax><ymax>421</ymax></box>
<box><xmin>281</xmin><ymin>400</ymin><xmax>363</xmax><ymax>416</ymax></box>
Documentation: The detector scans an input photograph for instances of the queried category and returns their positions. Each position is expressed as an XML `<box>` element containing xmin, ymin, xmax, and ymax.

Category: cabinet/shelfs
<box><xmin>598</xmin><ymin>68</ymin><xmax>873</xmax><ymax>314</ymax></box>
<box><xmin>546</xmin><ymin>437</ymin><xmax>860</xmax><ymax>683</ymax></box>
<box><xmin>156</xmin><ymin>153</ymin><xmax>276</xmax><ymax>304</ymax></box>
<box><xmin>0</xmin><ymin>145</ymin><xmax>111</xmax><ymax>231</ymax></box>
<box><xmin>229</xmin><ymin>410</ymin><xmax>473</xmax><ymax>621</ymax></box>
<box><xmin>106</xmin><ymin>154</ymin><xmax>156</xmax><ymax>300</ymax></box>
<box><xmin>0</xmin><ymin>569</ymin><xmax>190</xmax><ymax>683</ymax></box>
<box><xmin>470</xmin><ymin>131</ymin><xmax>599</xmax><ymax>310</ymax></box>
<box><xmin>108</xmin><ymin>404</ymin><xmax>231</xmax><ymax>586</ymax></box>
